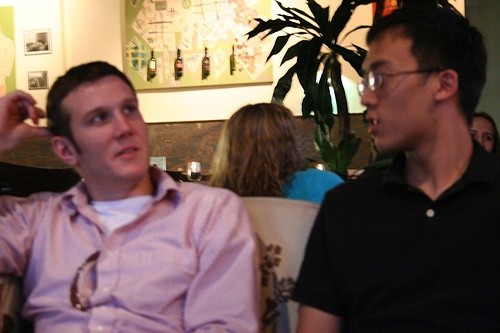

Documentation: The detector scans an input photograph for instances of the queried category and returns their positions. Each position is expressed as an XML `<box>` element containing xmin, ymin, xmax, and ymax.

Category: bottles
<box><xmin>147</xmin><ymin>50</ymin><xmax>157</xmax><ymax>81</ymax></box>
<box><xmin>230</xmin><ymin>45</ymin><xmax>237</xmax><ymax>75</ymax></box>
<box><xmin>202</xmin><ymin>47</ymin><xmax>210</xmax><ymax>80</ymax></box>
<box><xmin>174</xmin><ymin>49</ymin><xmax>183</xmax><ymax>80</ymax></box>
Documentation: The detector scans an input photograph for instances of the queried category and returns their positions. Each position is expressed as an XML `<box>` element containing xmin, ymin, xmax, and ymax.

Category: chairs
<box><xmin>240</xmin><ymin>197</ymin><xmax>320</xmax><ymax>333</ymax></box>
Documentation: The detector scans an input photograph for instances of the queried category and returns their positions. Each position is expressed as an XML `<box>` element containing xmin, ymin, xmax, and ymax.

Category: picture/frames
<box><xmin>27</xmin><ymin>71</ymin><xmax>49</xmax><ymax>90</ymax></box>
<box><xmin>24</xmin><ymin>29</ymin><xmax>52</xmax><ymax>55</ymax></box>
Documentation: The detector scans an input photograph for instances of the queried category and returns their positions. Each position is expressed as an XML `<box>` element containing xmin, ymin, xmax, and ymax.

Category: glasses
<box><xmin>357</xmin><ymin>69</ymin><xmax>439</xmax><ymax>97</ymax></box>
<box><xmin>70</xmin><ymin>251</ymin><xmax>101</xmax><ymax>312</ymax></box>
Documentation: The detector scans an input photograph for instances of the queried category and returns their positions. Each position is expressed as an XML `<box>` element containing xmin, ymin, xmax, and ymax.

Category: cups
<box><xmin>186</xmin><ymin>162</ymin><xmax>202</xmax><ymax>181</ymax></box>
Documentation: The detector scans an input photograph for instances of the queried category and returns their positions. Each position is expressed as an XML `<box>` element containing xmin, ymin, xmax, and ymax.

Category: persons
<box><xmin>290</xmin><ymin>1</ymin><xmax>500</xmax><ymax>333</ymax></box>
<box><xmin>208</xmin><ymin>101</ymin><xmax>345</xmax><ymax>205</ymax></box>
<box><xmin>469</xmin><ymin>112</ymin><xmax>498</xmax><ymax>151</ymax></box>
<box><xmin>0</xmin><ymin>60</ymin><xmax>262</xmax><ymax>333</ymax></box>
